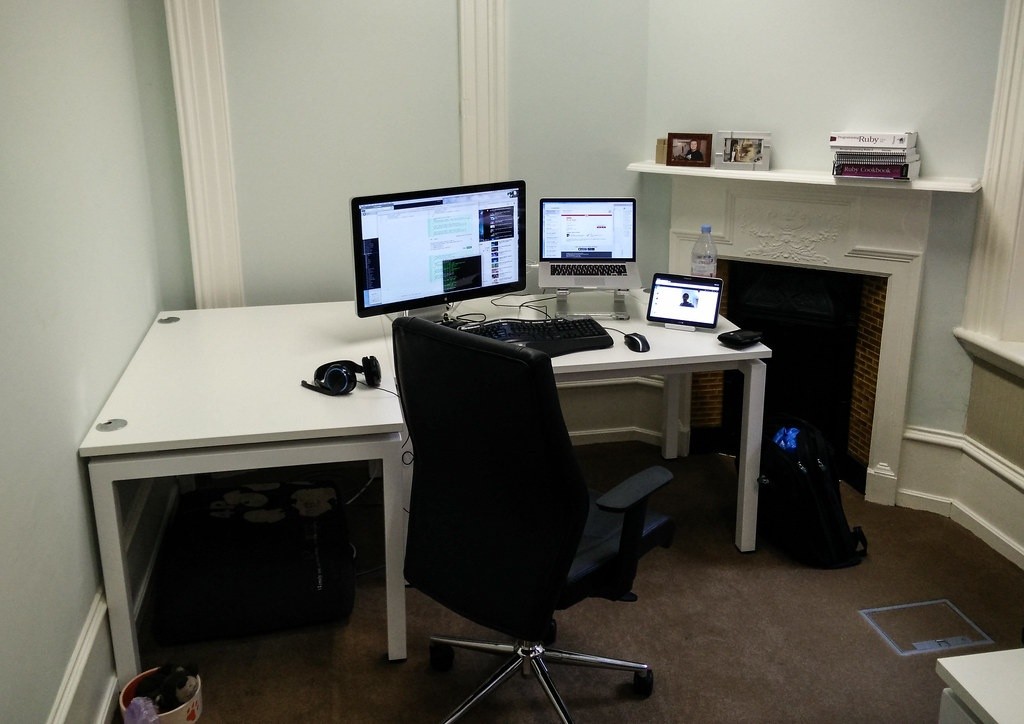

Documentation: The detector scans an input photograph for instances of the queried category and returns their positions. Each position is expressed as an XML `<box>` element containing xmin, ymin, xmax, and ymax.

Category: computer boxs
<box><xmin>179</xmin><ymin>479</ymin><xmax>357</xmax><ymax>642</ymax></box>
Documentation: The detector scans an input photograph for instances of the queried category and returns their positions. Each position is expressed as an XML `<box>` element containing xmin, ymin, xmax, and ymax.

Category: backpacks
<box><xmin>735</xmin><ymin>412</ymin><xmax>868</xmax><ymax>570</ymax></box>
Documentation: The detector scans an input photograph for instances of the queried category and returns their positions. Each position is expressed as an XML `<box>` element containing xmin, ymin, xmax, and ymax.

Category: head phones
<box><xmin>314</xmin><ymin>355</ymin><xmax>382</xmax><ymax>395</ymax></box>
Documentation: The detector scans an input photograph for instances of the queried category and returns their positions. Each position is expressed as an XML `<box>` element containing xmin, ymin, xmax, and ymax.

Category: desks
<box><xmin>437</xmin><ymin>285</ymin><xmax>773</xmax><ymax>551</ymax></box>
<box><xmin>76</xmin><ymin>299</ymin><xmax>415</xmax><ymax>697</ymax></box>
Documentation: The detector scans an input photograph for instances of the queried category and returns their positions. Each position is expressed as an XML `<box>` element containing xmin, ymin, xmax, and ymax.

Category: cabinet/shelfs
<box><xmin>935</xmin><ymin>647</ymin><xmax>1024</xmax><ymax>724</ymax></box>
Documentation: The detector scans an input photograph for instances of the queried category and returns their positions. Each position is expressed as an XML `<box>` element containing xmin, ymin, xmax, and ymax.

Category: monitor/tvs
<box><xmin>351</xmin><ymin>180</ymin><xmax>527</xmax><ymax>329</ymax></box>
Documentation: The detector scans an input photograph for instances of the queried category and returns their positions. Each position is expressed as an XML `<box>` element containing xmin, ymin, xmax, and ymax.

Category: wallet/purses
<box><xmin>718</xmin><ymin>330</ymin><xmax>763</xmax><ymax>350</ymax></box>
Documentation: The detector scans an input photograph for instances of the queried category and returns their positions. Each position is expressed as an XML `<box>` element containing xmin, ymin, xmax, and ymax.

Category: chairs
<box><xmin>392</xmin><ymin>318</ymin><xmax>675</xmax><ymax>724</ymax></box>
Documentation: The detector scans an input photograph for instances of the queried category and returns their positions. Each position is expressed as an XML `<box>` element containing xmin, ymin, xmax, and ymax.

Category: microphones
<box><xmin>301</xmin><ymin>380</ymin><xmax>336</xmax><ymax>396</ymax></box>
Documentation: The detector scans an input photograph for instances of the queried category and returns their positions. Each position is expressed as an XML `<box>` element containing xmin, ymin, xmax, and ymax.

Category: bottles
<box><xmin>690</xmin><ymin>225</ymin><xmax>717</xmax><ymax>277</ymax></box>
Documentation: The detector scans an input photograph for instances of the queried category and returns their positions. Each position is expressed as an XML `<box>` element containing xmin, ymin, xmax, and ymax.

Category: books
<box><xmin>828</xmin><ymin>131</ymin><xmax>921</xmax><ymax>181</ymax></box>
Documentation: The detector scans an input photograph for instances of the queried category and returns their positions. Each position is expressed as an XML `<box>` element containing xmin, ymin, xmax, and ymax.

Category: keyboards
<box><xmin>459</xmin><ymin>319</ymin><xmax>613</xmax><ymax>357</ymax></box>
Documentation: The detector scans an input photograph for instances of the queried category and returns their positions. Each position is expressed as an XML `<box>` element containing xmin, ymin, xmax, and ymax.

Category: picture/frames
<box><xmin>667</xmin><ymin>133</ymin><xmax>712</xmax><ymax>168</ymax></box>
<box><xmin>715</xmin><ymin>131</ymin><xmax>771</xmax><ymax>170</ymax></box>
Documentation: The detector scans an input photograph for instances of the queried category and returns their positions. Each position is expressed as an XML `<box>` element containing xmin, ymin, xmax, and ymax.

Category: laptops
<box><xmin>538</xmin><ymin>197</ymin><xmax>642</xmax><ymax>290</ymax></box>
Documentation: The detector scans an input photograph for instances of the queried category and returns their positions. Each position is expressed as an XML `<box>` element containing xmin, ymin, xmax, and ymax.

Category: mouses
<box><xmin>624</xmin><ymin>332</ymin><xmax>650</xmax><ymax>352</ymax></box>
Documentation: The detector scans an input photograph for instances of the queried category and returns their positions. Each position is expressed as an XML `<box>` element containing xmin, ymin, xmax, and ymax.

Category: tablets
<box><xmin>646</xmin><ymin>272</ymin><xmax>724</xmax><ymax>330</ymax></box>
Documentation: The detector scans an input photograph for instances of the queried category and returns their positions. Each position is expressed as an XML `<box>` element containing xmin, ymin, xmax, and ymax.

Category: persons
<box><xmin>680</xmin><ymin>294</ymin><xmax>694</xmax><ymax>307</ymax></box>
<box><xmin>685</xmin><ymin>140</ymin><xmax>704</xmax><ymax>161</ymax></box>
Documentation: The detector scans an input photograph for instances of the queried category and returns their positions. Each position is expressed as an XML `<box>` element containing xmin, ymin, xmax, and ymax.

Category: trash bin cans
<box><xmin>118</xmin><ymin>662</ymin><xmax>203</xmax><ymax>724</ymax></box>
